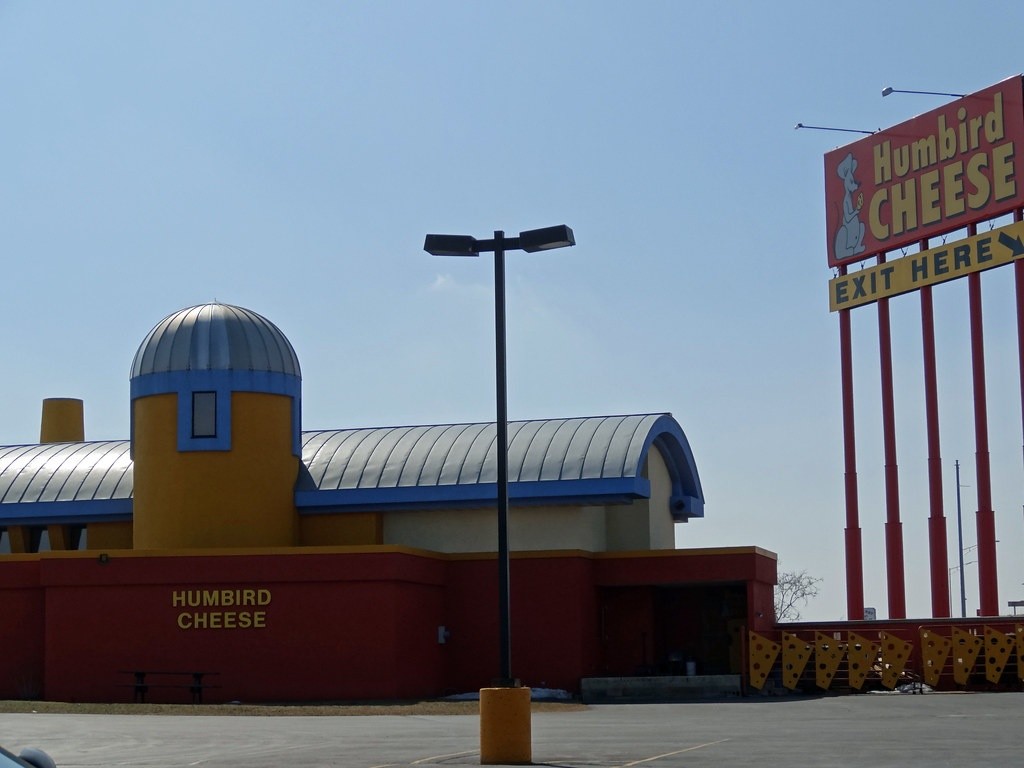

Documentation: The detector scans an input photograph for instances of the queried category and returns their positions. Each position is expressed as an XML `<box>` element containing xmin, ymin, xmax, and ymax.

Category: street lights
<box><xmin>424</xmin><ymin>223</ymin><xmax>577</xmax><ymax>688</ymax></box>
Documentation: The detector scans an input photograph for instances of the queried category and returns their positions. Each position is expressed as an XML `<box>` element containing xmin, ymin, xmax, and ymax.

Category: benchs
<box><xmin>113</xmin><ymin>668</ymin><xmax>225</xmax><ymax>705</ymax></box>
